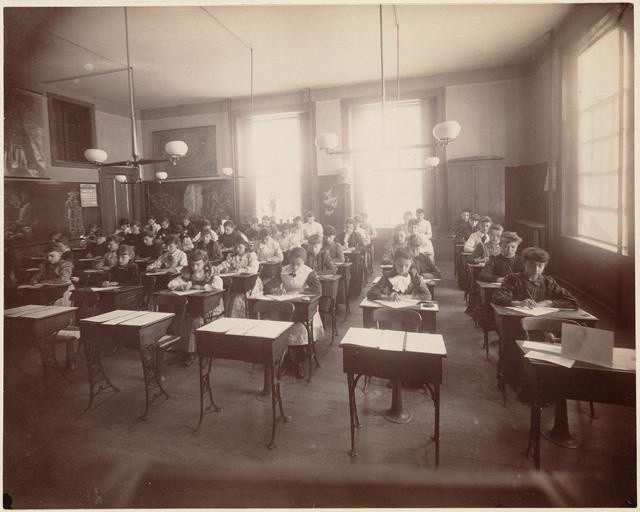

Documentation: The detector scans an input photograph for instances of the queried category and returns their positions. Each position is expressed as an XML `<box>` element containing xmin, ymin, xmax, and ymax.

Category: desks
<box><xmin>4</xmin><ymin>230</ymin><xmax>636</xmax><ymax>472</ymax></box>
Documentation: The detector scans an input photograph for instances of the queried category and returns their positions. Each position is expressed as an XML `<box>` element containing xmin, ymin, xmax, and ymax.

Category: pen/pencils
<box><xmin>509</xmin><ymin>265</ymin><xmax>513</xmax><ymax>273</ymax></box>
<box><xmin>527</xmin><ymin>289</ymin><xmax>533</xmax><ymax>300</ymax></box>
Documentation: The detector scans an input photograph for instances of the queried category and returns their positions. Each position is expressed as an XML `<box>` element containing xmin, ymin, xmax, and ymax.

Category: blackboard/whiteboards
<box><xmin>152</xmin><ymin>125</ymin><xmax>220</xmax><ymax>180</ymax></box>
<box><xmin>4</xmin><ymin>180</ymin><xmax>103</xmax><ymax>250</ymax></box>
<box><xmin>146</xmin><ymin>180</ymin><xmax>235</xmax><ymax>228</ymax></box>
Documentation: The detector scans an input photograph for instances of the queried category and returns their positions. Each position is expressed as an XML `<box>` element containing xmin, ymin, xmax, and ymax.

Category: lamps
<box><xmin>424</xmin><ymin>155</ymin><xmax>440</xmax><ymax>171</ymax></box>
<box><xmin>84</xmin><ymin>9</ymin><xmax>191</xmax><ymax>169</ymax></box>
<box><xmin>220</xmin><ymin>166</ymin><xmax>234</xmax><ymax>177</ymax></box>
<box><xmin>315</xmin><ymin>4</ymin><xmax>463</xmax><ymax>155</ymax></box>
<box><xmin>112</xmin><ymin>67</ymin><xmax>168</xmax><ymax>184</ymax></box>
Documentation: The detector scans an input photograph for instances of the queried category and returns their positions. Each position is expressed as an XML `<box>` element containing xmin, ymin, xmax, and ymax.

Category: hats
<box><xmin>520</xmin><ymin>247</ymin><xmax>550</xmax><ymax>263</ymax></box>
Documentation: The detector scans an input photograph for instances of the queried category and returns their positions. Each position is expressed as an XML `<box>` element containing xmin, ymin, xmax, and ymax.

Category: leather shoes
<box><xmin>281</xmin><ymin>362</ymin><xmax>306</xmax><ymax>378</ymax></box>
<box><xmin>177</xmin><ymin>352</ymin><xmax>195</xmax><ymax>367</ymax></box>
